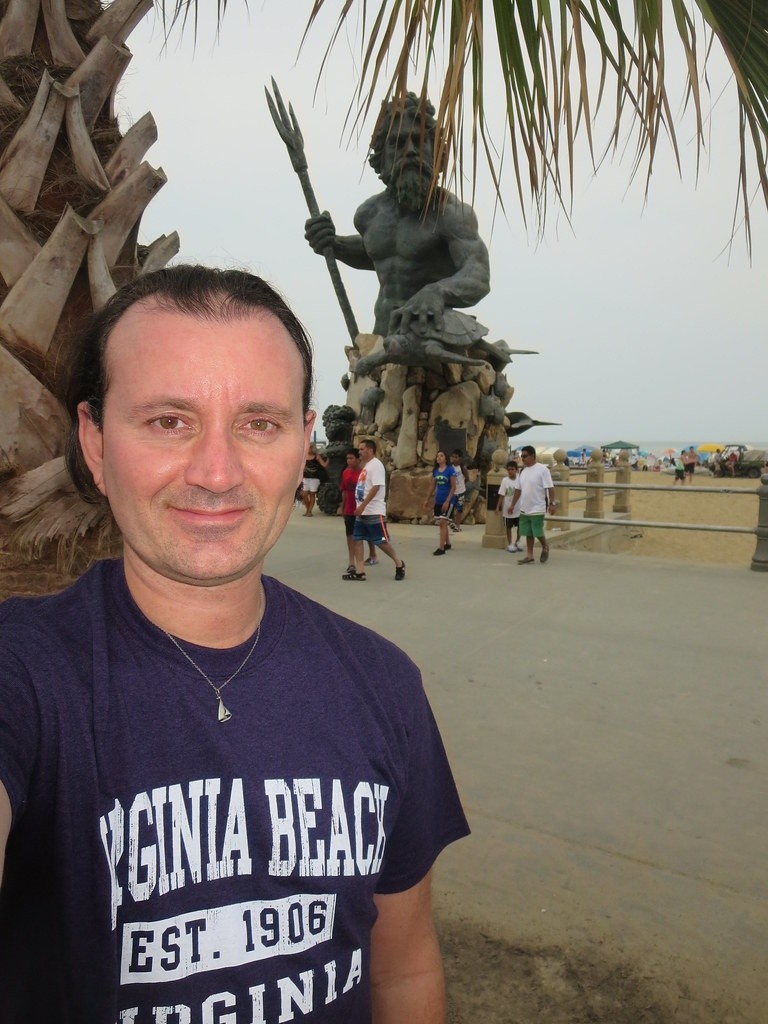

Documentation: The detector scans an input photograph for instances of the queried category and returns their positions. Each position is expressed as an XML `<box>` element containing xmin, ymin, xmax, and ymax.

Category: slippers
<box><xmin>347</xmin><ymin>565</ymin><xmax>357</xmax><ymax>573</ymax></box>
<box><xmin>453</xmin><ymin>528</ymin><xmax>462</xmax><ymax>532</ymax></box>
<box><xmin>363</xmin><ymin>557</ymin><xmax>379</xmax><ymax>565</ymax></box>
<box><xmin>450</xmin><ymin>523</ymin><xmax>456</xmax><ymax>529</ymax></box>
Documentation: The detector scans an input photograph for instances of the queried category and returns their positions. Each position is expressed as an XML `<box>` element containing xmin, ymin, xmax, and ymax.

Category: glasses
<box><xmin>521</xmin><ymin>453</ymin><xmax>530</xmax><ymax>458</ymax></box>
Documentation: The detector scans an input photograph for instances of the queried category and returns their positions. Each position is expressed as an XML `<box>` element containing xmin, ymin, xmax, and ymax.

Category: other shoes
<box><xmin>434</xmin><ymin>548</ymin><xmax>445</xmax><ymax>555</ymax></box>
<box><xmin>518</xmin><ymin>557</ymin><xmax>534</xmax><ymax>563</ymax></box>
<box><xmin>507</xmin><ymin>545</ymin><xmax>517</xmax><ymax>552</ymax></box>
<box><xmin>445</xmin><ymin>544</ymin><xmax>451</xmax><ymax>549</ymax></box>
<box><xmin>515</xmin><ymin>540</ymin><xmax>524</xmax><ymax>551</ymax></box>
<box><xmin>540</xmin><ymin>545</ymin><xmax>549</xmax><ymax>562</ymax></box>
<box><xmin>342</xmin><ymin>570</ymin><xmax>367</xmax><ymax>581</ymax></box>
<box><xmin>395</xmin><ymin>560</ymin><xmax>406</xmax><ymax>580</ymax></box>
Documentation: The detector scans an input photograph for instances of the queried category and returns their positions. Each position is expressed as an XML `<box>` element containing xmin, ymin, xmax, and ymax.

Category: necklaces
<box><xmin>146</xmin><ymin>582</ymin><xmax>266</xmax><ymax>722</ymax></box>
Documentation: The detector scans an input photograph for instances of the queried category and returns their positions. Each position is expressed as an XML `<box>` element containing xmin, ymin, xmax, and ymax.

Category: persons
<box><xmin>423</xmin><ymin>450</ymin><xmax>469</xmax><ymax>556</ymax></box>
<box><xmin>304</xmin><ymin>91</ymin><xmax>491</xmax><ymax>334</ymax></box>
<box><xmin>579</xmin><ymin>448</ymin><xmax>587</xmax><ymax>468</ymax></box>
<box><xmin>708</xmin><ymin>449</ymin><xmax>738</xmax><ymax>477</ymax></box>
<box><xmin>494</xmin><ymin>446</ymin><xmax>553</xmax><ymax>563</ymax></box>
<box><xmin>337</xmin><ymin>439</ymin><xmax>406</xmax><ymax>580</ymax></box>
<box><xmin>600</xmin><ymin>449</ymin><xmax>619</xmax><ymax>471</ymax></box>
<box><xmin>302</xmin><ymin>441</ymin><xmax>330</xmax><ymax>517</ymax></box>
<box><xmin>1</xmin><ymin>268</ymin><xmax>469</xmax><ymax>1023</ymax></box>
<box><xmin>630</xmin><ymin>451</ymin><xmax>672</xmax><ymax>472</ymax></box>
<box><xmin>673</xmin><ymin>446</ymin><xmax>697</xmax><ymax>485</ymax></box>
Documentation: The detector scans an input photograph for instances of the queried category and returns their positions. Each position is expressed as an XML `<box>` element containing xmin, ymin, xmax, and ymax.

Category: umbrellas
<box><xmin>697</xmin><ymin>442</ymin><xmax>726</xmax><ymax>452</ymax></box>
